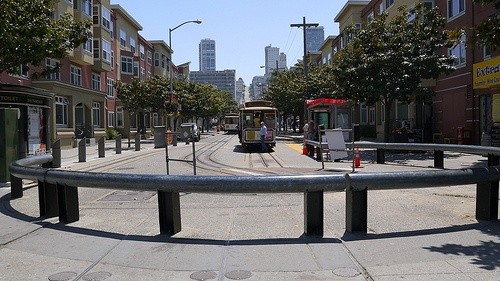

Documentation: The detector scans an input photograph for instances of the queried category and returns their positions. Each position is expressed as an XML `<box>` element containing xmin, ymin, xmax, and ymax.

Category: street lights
<box><xmin>169</xmin><ymin>20</ymin><xmax>202</xmax><ymax>105</ymax></box>
<box><xmin>260</xmin><ymin>66</ymin><xmax>278</xmax><ymax>80</ymax></box>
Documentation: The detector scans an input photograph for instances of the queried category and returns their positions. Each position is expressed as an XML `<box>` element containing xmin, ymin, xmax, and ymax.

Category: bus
<box><xmin>224</xmin><ymin>113</ymin><xmax>241</xmax><ymax>135</ymax></box>
<box><xmin>237</xmin><ymin>99</ymin><xmax>277</xmax><ymax>149</ymax></box>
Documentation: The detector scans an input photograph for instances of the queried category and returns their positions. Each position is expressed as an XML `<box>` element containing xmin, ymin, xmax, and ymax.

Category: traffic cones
<box><xmin>351</xmin><ymin>146</ymin><xmax>365</xmax><ymax>168</ymax></box>
<box><xmin>302</xmin><ymin>140</ymin><xmax>309</xmax><ymax>155</ymax></box>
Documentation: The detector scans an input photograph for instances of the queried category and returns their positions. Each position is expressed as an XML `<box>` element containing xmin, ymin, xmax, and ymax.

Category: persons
<box><xmin>396</xmin><ymin>121</ymin><xmax>408</xmax><ymax>164</ymax></box>
<box><xmin>302</xmin><ymin>120</ymin><xmax>316</xmax><ymax>158</ymax></box>
<box><xmin>259</xmin><ymin>121</ymin><xmax>272</xmax><ymax>153</ymax></box>
